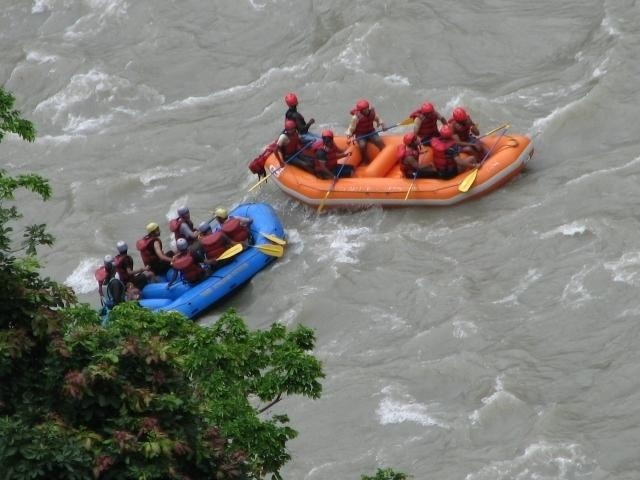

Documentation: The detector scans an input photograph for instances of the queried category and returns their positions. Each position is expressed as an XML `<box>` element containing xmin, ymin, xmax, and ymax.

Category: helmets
<box><xmin>357</xmin><ymin>100</ymin><xmax>370</xmax><ymax>113</ymax></box>
<box><xmin>404</xmin><ymin>103</ymin><xmax>467</xmax><ymax>146</ymax></box>
<box><xmin>176</xmin><ymin>205</ymin><xmax>228</xmax><ymax>251</ymax></box>
<box><xmin>104</xmin><ymin>255</ymin><xmax>114</xmax><ymax>266</ymax></box>
<box><xmin>284</xmin><ymin>120</ymin><xmax>297</xmax><ymax>131</ymax></box>
<box><xmin>322</xmin><ymin>130</ymin><xmax>333</xmax><ymax>139</ymax></box>
<box><xmin>147</xmin><ymin>223</ymin><xmax>159</xmax><ymax>235</ymax></box>
<box><xmin>117</xmin><ymin>240</ymin><xmax>128</xmax><ymax>254</ymax></box>
<box><xmin>285</xmin><ymin>93</ymin><xmax>298</xmax><ymax>107</ymax></box>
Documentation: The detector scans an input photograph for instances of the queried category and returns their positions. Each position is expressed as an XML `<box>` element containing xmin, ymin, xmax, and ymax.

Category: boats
<box><xmin>264</xmin><ymin>133</ymin><xmax>533</xmax><ymax>210</ymax></box>
<box><xmin>101</xmin><ymin>202</ymin><xmax>284</xmax><ymax>321</ymax></box>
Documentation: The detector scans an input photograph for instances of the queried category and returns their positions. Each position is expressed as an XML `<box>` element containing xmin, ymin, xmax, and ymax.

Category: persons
<box><xmin>277</xmin><ymin>94</ymin><xmax>482</xmax><ymax>180</ymax></box>
<box><xmin>105</xmin><ymin>205</ymin><xmax>253</xmax><ymax>304</ymax></box>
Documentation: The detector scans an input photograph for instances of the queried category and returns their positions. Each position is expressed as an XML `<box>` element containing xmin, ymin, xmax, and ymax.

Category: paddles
<box><xmin>246</xmin><ymin>244</ymin><xmax>284</xmax><ymax>258</ymax></box>
<box><xmin>251</xmin><ymin>228</ymin><xmax>286</xmax><ymax>245</ymax></box>
<box><xmin>459</xmin><ymin>124</ymin><xmax>510</xmax><ymax>193</ymax></box>
<box><xmin>249</xmin><ymin>139</ymin><xmax>316</xmax><ymax>193</ymax></box>
<box><xmin>348</xmin><ymin>117</ymin><xmax>414</xmax><ymax>145</ymax></box>
<box><xmin>317</xmin><ymin>152</ymin><xmax>352</xmax><ymax>213</ymax></box>
<box><xmin>215</xmin><ymin>243</ymin><xmax>243</xmax><ymax>260</ymax></box>
